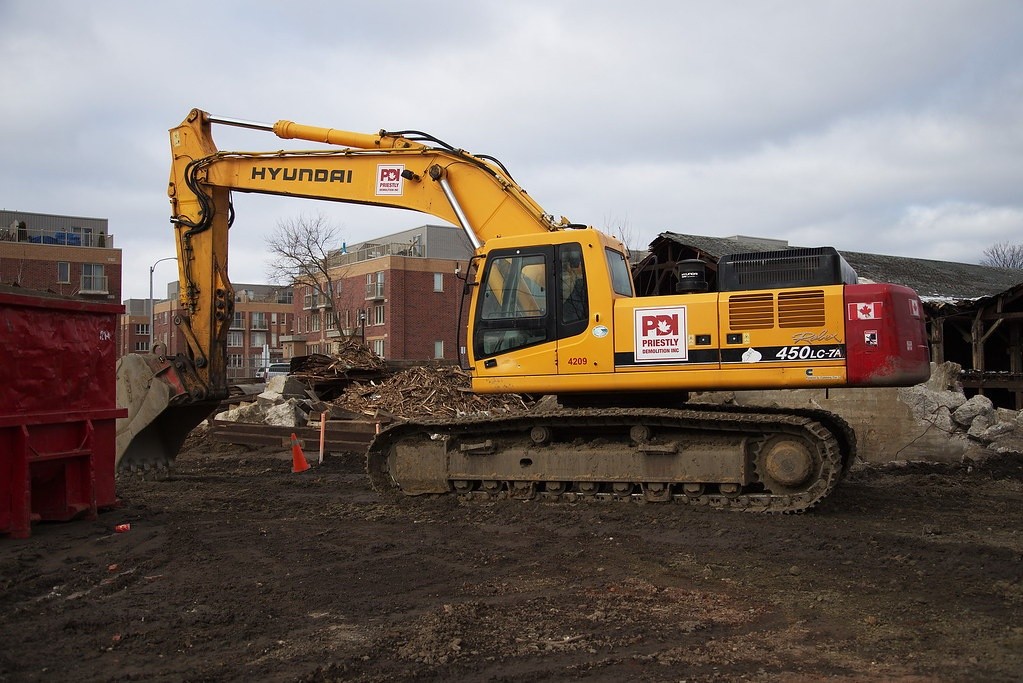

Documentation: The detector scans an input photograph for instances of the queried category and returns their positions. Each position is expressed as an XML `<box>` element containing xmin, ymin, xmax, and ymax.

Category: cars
<box><xmin>255</xmin><ymin>367</ymin><xmax>266</xmax><ymax>378</ymax></box>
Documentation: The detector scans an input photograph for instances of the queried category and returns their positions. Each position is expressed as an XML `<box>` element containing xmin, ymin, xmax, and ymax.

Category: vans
<box><xmin>265</xmin><ymin>363</ymin><xmax>290</xmax><ymax>380</ymax></box>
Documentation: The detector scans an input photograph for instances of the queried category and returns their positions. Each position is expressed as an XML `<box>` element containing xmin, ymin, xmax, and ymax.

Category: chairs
<box><xmin>563</xmin><ymin>279</ymin><xmax>589</xmax><ymax>323</ymax></box>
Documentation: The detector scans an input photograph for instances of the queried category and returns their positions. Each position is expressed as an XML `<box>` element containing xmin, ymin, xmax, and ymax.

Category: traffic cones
<box><xmin>289</xmin><ymin>432</ymin><xmax>312</xmax><ymax>473</ymax></box>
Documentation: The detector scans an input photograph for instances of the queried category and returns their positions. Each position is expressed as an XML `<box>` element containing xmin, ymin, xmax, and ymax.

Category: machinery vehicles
<box><xmin>109</xmin><ymin>108</ymin><xmax>931</xmax><ymax>512</ymax></box>
